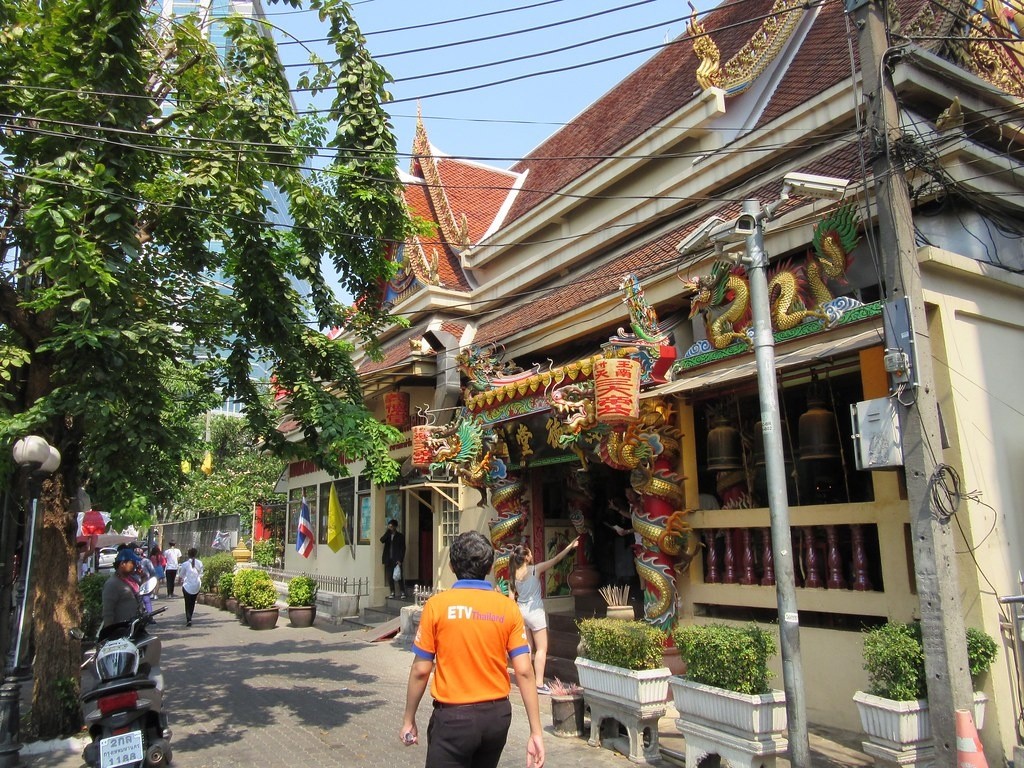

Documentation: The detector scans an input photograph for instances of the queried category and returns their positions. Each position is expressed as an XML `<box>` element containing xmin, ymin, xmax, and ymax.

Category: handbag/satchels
<box><xmin>393</xmin><ymin>564</ymin><xmax>401</xmax><ymax>581</ymax></box>
<box><xmin>154</xmin><ymin>565</ymin><xmax>165</xmax><ymax>580</ymax></box>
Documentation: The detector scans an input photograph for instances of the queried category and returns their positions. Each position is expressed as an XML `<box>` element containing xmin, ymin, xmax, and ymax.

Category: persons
<box><xmin>380</xmin><ymin>519</ymin><xmax>406</xmax><ymax>599</ymax></box>
<box><xmin>399</xmin><ymin>530</ymin><xmax>546</xmax><ymax>768</ymax></box>
<box><xmin>179</xmin><ymin>548</ymin><xmax>203</xmax><ymax>626</ymax></box>
<box><xmin>164</xmin><ymin>540</ymin><xmax>182</xmax><ymax>598</ymax></box>
<box><xmin>118</xmin><ymin>543</ymin><xmax>139</xmax><ymax>550</ymax></box>
<box><xmin>150</xmin><ymin>547</ymin><xmax>166</xmax><ymax>600</ymax></box>
<box><xmin>134</xmin><ymin>548</ymin><xmax>156</xmax><ymax>624</ymax></box>
<box><xmin>609</xmin><ymin>485</ymin><xmax>645</xmax><ymax>593</ymax></box>
<box><xmin>77</xmin><ymin>540</ymin><xmax>95</xmax><ymax>581</ymax></box>
<box><xmin>509</xmin><ymin>536</ymin><xmax>580</xmax><ymax>694</ymax></box>
<box><xmin>102</xmin><ymin>550</ymin><xmax>143</xmax><ymax>625</ymax></box>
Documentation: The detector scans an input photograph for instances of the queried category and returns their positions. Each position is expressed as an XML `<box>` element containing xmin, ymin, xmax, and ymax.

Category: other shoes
<box><xmin>537</xmin><ymin>683</ymin><xmax>551</xmax><ymax>694</ymax></box>
<box><xmin>401</xmin><ymin>594</ymin><xmax>406</xmax><ymax>599</ymax></box>
<box><xmin>389</xmin><ymin>594</ymin><xmax>395</xmax><ymax>598</ymax></box>
<box><xmin>184</xmin><ymin>621</ymin><xmax>192</xmax><ymax>628</ymax></box>
<box><xmin>150</xmin><ymin>594</ymin><xmax>153</xmax><ymax>598</ymax></box>
<box><xmin>166</xmin><ymin>594</ymin><xmax>172</xmax><ymax>598</ymax></box>
<box><xmin>149</xmin><ymin>619</ymin><xmax>156</xmax><ymax>624</ymax></box>
<box><xmin>154</xmin><ymin>595</ymin><xmax>158</xmax><ymax>599</ymax></box>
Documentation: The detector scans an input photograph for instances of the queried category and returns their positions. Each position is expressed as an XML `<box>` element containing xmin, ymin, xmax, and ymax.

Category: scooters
<box><xmin>68</xmin><ymin>603</ymin><xmax>174</xmax><ymax>768</ymax></box>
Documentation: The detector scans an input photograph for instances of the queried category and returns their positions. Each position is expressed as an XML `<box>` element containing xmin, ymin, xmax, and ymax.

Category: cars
<box><xmin>88</xmin><ymin>539</ymin><xmax>159</xmax><ymax>570</ymax></box>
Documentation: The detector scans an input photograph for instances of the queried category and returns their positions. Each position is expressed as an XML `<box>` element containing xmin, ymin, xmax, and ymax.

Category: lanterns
<box><xmin>383</xmin><ymin>391</ymin><xmax>412</xmax><ymax>426</ymax></box>
<box><xmin>411</xmin><ymin>425</ymin><xmax>438</xmax><ymax>466</ymax></box>
<box><xmin>594</xmin><ymin>359</ymin><xmax>640</xmax><ymax>423</ymax></box>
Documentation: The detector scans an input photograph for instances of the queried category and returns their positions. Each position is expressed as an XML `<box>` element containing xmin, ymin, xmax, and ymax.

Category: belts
<box><xmin>441</xmin><ymin>697</ymin><xmax>508</xmax><ymax>707</ymax></box>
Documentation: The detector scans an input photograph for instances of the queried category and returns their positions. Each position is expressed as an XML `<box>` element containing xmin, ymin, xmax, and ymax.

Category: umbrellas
<box><xmin>77</xmin><ymin>510</ymin><xmax>138</xmax><ymax>538</ymax></box>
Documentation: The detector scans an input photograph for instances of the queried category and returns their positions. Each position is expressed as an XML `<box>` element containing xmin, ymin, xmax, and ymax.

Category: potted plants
<box><xmin>287</xmin><ymin>577</ymin><xmax>320</xmax><ymax>627</ymax></box>
<box><xmin>196</xmin><ymin>553</ymin><xmax>280</xmax><ymax>630</ymax></box>
<box><xmin>668</xmin><ymin>626</ymin><xmax>789</xmax><ymax>733</ymax></box>
<box><xmin>854</xmin><ymin>620</ymin><xmax>997</xmax><ymax>744</ymax></box>
<box><xmin>573</xmin><ymin>618</ymin><xmax>671</xmax><ymax>704</ymax></box>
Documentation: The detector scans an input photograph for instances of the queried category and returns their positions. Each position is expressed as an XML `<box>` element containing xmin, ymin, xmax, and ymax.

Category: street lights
<box><xmin>11</xmin><ymin>436</ymin><xmax>63</xmax><ymax>668</ymax></box>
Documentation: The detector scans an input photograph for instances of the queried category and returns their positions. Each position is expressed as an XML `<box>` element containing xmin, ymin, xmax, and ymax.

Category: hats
<box><xmin>115</xmin><ymin>549</ymin><xmax>146</xmax><ymax>561</ymax></box>
<box><xmin>152</xmin><ymin>541</ymin><xmax>157</xmax><ymax>547</ymax></box>
<box><xmin>134</xmin><ymin>548</ymin><xmax>144</xmax><ymax>555</ymax></box>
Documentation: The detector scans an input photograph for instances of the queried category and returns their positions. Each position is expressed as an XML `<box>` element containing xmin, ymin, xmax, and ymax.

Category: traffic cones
<box><xmin>955</xmin><ymin>708</ymin><xmax>989</xmax><ymax>768</ymax></box>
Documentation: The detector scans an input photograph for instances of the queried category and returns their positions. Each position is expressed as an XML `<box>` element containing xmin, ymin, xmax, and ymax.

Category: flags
<box><xmin>328</xmin><ymin>483</ymin><xmax>347</xmax><ymax>553</ymax></box>
<box><xmin>296</xmin><ymin>497</ymin><xmax>314</xmax><ymax>557</ymax></box>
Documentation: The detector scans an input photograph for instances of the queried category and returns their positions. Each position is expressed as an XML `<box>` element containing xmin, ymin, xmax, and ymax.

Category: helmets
<box><xmin>96</xmin><ymin>637</ymin><xmax>139</xmax><ymax>681</ymax></box>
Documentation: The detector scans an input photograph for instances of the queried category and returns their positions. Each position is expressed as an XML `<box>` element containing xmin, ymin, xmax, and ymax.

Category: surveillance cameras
<box><xmin>780</xmin><ymin>172</ymin><xmax>850</xmax><ymax>202</ymax></box>
<box><xmin>675</xmin><ymin>216</ymin><xmax>726</xmax><ymax>255</ymax></box>
<box><xmin>708</xmin><ymin>214</ymin><xmax>756</xmax><ymax>243</ymax></box>
